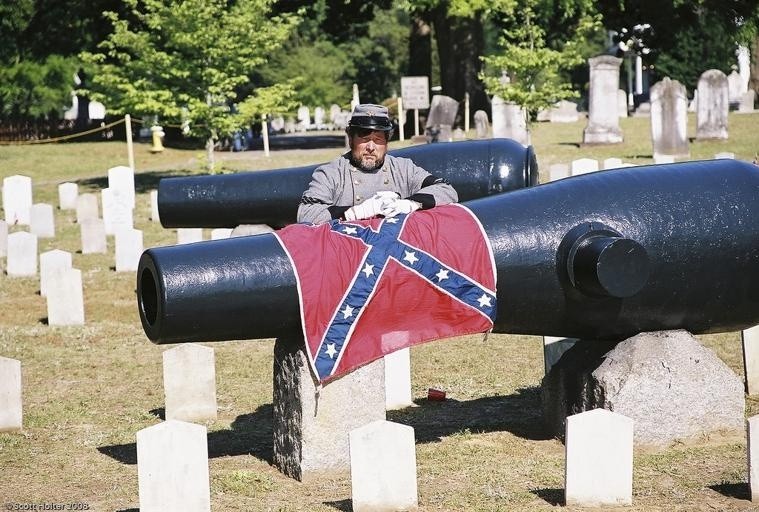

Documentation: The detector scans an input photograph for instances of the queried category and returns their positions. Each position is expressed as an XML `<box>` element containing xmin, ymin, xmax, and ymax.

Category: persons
<box><xmin>296</xmin><ymin>102</ymin><xmax>459</xmax><ymax>225</ymax></box>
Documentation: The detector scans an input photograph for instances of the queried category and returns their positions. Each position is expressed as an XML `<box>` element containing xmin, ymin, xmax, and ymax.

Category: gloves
<box><xmin>344</xmin><ymin>190</ymin><xmax>420</xmax><ymax>222</ymax></box>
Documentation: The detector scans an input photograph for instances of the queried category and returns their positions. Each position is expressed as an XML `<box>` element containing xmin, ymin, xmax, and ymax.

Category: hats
<box><xmin>346</xmin><ymin>104</ymin><xmax>393</xmax><ymax>130</ymax></box>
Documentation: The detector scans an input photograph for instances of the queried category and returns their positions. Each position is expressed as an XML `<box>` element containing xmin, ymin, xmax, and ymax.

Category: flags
<box><xmin>270</xmin><ymin>202</ymin><xmax>498</xmax><ymax>385</ymax></box>
